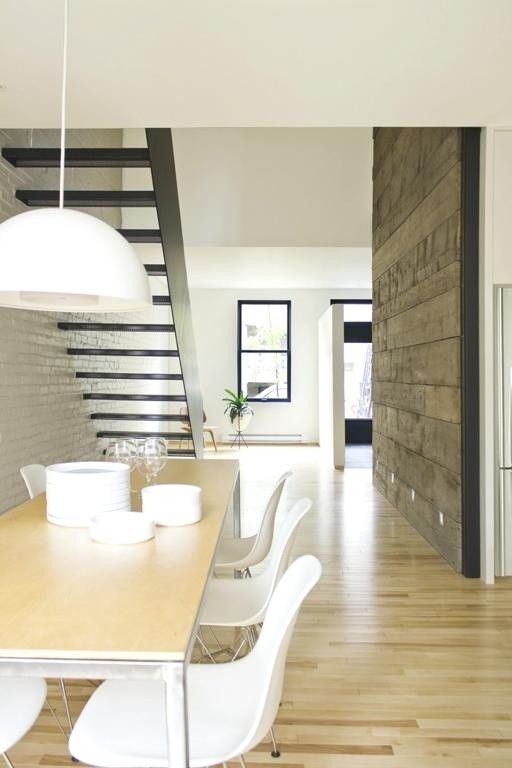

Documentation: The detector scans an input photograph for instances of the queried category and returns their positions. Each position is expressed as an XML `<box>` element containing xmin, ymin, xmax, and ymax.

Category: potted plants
<box><xmin>221</xmin><ymin>388</ymin><xmax>255</xmax><ymax>449</ymax></box>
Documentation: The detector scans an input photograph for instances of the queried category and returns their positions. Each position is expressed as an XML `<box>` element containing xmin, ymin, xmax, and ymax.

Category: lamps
<box><xmin>1</xmin><ymin>0</ymin><xmax>153</xmax><ymax>314</ymax></box>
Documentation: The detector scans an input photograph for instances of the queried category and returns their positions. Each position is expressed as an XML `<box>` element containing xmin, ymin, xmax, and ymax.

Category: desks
<box><xmin>0</xmin><ymin>457</ymin><xmax>241</xmax><ymax>768</ymax></box>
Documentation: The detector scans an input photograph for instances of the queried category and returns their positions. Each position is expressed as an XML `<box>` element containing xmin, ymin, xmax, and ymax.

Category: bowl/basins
<box><xmin>141</xmin><ymin>485</ymin><xmax>202</xmax><ymax>526</ymax></box>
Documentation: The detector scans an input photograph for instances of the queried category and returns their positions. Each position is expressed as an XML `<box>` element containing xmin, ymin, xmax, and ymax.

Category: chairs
<box><xmin>66</xmin><ymin>552</ymin><xmax>323</xmax><ymax>768</ymax></box>
<box><xmin>195</xmin><ymin>497</ymin><xmax>313</xmax><ymax>664</ymax></box>
<box><xmin>179</xmin><ymin>407</ymin><xmax>218</xmax><ymax>451</ymax></box>
<box><xmin>0</xmin><ymin>675</ymin><xmax>75</xmax><ymax>768</ymax></box>
<box><xmin>213</xmin><ymin>471</ymin><xmax>293</xmax><ymax>576</ymax></box>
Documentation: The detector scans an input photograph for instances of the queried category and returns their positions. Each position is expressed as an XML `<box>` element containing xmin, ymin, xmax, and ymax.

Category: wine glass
<box><xmin>105</xmin><ymin>438</ymin><xmax>170</xmax><ymax>494</ymax></box>
